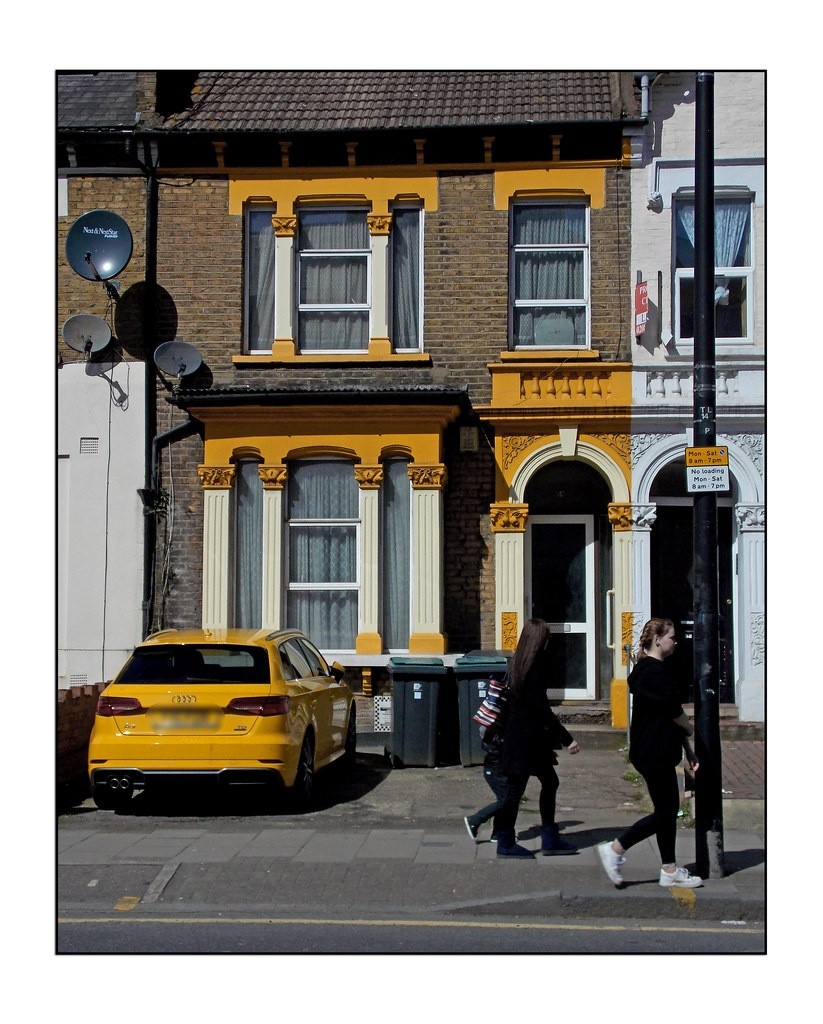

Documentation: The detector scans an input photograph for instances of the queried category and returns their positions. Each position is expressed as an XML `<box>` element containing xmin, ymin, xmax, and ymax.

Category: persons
<box><xmin>463</xmin><ymin>619</ymin><xmax>580</xmax><ymax>859</ymax></box>
<box><xmin>598</xmin><ymin>618</ymin><xmax>702</xmax><ymax>887</ymax></box>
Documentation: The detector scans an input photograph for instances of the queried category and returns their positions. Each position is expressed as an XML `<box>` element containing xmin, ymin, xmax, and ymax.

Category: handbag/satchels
<box><xmin>476</xmin><ymin>672</ymin><xmax>514</xmax><ymax>734</ymax></box>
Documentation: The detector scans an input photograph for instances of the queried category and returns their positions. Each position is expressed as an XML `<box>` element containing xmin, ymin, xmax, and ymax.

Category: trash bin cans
<box><xmin>386</xmin><ymin>657</ymin><xmax>448</xmax><ymax>768</ymax></box>
<box><xmin>455</xmin><ymin>651</ymin><xmax>508</xmax><ymax>767</ymax></box>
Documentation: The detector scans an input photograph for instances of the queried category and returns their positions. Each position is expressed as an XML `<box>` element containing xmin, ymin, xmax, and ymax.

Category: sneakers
<box><xmin>598</xmin><ymin>841</ymin><xmax>628</xmax><ymax>885</ymax></box>
<box><xmin>658</xmin><ymin>867</ymin><xmax>703</xmax><ymax>888</ymax></box>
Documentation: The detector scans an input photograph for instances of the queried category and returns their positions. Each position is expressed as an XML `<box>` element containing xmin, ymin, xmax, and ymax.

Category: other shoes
<box><xmin>496</xmin><ymin>844</ymin><xmax>535</xmax><ymax>859</ymax></box>
<box><xmin>464</xmin><ymin>815</ymin><xmax>481</xmax><ymax>840</ymax></box>
<box><xmin>490</xmin><ymin>835</ymin><xmax>518</xmax><ymax>843</ymax></box>
<box><xmin>541</xmin><ymin>839</ymin><xmax>578</xmax><ymax>856</ymax></box>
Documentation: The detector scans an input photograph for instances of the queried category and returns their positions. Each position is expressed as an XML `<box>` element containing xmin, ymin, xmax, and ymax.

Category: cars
<box><xmin>86</xmin><ymin>629</ymin><xmax>362</xmax><ymax>810</ymax></box>
